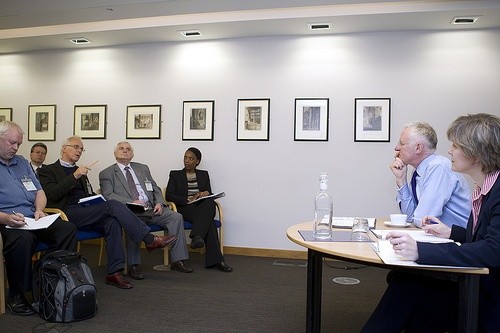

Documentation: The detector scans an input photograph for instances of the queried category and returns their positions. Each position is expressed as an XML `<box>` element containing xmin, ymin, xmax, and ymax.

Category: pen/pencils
<box><xmin>90</xmin><ymin>158</ymin><xmax>99</xmax><ymax>169</ymax></box>
<box><xmin>11</xmin><ymin>209</ymin><xmax>30</xmax><ymax>228</ymax></box>
<box><xmin>426</xmin><ymin>219</ymin><xmax>439</xmax><ymax>225</ymax></box>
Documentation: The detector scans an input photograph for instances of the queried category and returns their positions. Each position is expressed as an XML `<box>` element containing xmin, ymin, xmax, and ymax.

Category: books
<box><xmin>187</xmin><ymin>192</ymin><xmax>225</xmax><ymax>205</ymax></box>
<box><xmin>126</xmin><ymin>202</ymin><xmax>148</xmax><ymax>212</ymax></box>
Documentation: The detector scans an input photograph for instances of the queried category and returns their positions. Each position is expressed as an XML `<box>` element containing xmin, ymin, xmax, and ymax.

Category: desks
<box><xmin>287</xmin><ymin>219</ymin><xmax>489</xmax><ymax>333</ymax></box>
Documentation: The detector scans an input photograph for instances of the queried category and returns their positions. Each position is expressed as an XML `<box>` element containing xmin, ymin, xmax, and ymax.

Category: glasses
<box><xmin>64</xmin><ymin>144</ymin><xmax>86</xmax><ymax>151</ymax></box>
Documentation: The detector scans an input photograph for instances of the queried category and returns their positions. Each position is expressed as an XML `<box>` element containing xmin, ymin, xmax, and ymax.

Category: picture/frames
<box><xmin>125</xmin><ymin>105</ymin><xmax>162</xmax><ymax>140</ymax></box>
<box><xmin>354</xmin><ymin>98</ymin><xmax>390</xmax><ymax>143</ymax></box>
<box><xmin>28</xmin><ymin>105</ymin><xmax>57</xmax><ymax>141</ymax></box>
<box><xmin>73</xmin><ymin>104</ymin><xmax>107</xmax><ymax>139</ymax></box>
<box><xmin>236</xmin><ymin>99</ymin><xmax>271</xmax><ymax>141</ymax></box>
<box><xmin>0</xmin><ymin>108</ymin><xmax>13</xmax><ymax>123</ymax></box>
<box><xmin>182</xmin><ymin>100</ymin><xmax>215</xmax><ymax>141</ymax></box>
<box><xmin>294</xmin><ymin>98</ymin><xmax>329</xmax><ymax>141</ymax></box>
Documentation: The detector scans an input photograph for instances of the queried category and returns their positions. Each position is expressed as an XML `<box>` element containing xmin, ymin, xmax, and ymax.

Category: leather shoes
<box><xmin>7</xmin><ymin>292</ymin><xmax>34</xmax><ymax>315</ymax></box>
<box><xmin>105</xmin><ymin>273</ymin><xmax>133</xmax><ymax>289</ymax></box>
<box><xmin>169</xmin><ymin>259</ymin><xmax>193</xmax><ymax>273</ymax></box>
<box><xmin>128</xmin><ymin>264</ymin><xmax>145</xmax><ymax>280</ymax></box>
<box><xmin>146</xmin><ymin>234</ymin><xmax>178</xmax><ymax>251</ymax></box>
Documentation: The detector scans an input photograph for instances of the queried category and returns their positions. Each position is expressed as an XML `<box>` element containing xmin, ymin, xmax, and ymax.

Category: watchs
<box><xmin>161</xmin><ymin>204</ymin><xmax>164</xmax><ymax>208</ymax></box>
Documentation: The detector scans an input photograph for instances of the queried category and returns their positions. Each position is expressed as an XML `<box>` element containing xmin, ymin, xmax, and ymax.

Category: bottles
<box><xmin>314</xmin><ymin>173</ymin><xmax>333</xmax><ymax>239</ymax></box>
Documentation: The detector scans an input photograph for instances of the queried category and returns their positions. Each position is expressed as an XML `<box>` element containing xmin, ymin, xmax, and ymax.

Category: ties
<box><xmin>411</xmin><ymin>170</ymin><xmax>419</xmax><ymax>207</ymax></box>
<box><xmin>123</xmin><ymin>166</ymin><xmax>139</xmax><ymax>201</ymax></box>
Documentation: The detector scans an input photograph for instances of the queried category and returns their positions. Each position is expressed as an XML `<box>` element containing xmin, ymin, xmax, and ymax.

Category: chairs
<box><xmin>43</xmin><ymin>207</ymin><xmax>105</xmax><ymax>266</ymax></box>
<box><xmin>0</xmin><ymin>231</ymin><xmax>50</xmax><ymax>314</ymax></box>
<box><xmin>96</xmin><ymin>188</ymin><xmax>169</xmax><ymax>274</ymax></box>
<box><xmin>163</xmin><ymin>188</ymin><xmax>224</xmax><ymax>256</ymax></box>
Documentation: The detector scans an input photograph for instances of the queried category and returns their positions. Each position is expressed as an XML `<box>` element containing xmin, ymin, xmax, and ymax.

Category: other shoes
<box><xmin>217</xmin><ymin>260</ymin><xmax>233</xmax><ymax>272</ymax></box>
<box><xmin>190</xmin><ymin>235</ymin><xmax>204</xmax><ymax>249</ymax></box>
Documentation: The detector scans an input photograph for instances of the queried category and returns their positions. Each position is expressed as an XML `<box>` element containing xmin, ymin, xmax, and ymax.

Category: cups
<box><xmin>390</xmin><ymin>213</ymin><xmax>407</xmax><ymax>224</ymax></box>
<box><xmin>350</xmin><ymin>218</ymin><xmax>370</xmax><ymax>241</ymax></box>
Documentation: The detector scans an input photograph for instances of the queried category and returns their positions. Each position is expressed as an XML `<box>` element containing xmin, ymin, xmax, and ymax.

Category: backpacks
<box><xmin>32</xmin><ymin>249</ymin><xmax>98</xmax><ymax>323</ymax></box>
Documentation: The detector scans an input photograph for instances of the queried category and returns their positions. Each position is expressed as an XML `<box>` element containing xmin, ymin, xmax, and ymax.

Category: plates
<box><xmin>384</xmin><ymin>221</ymin><xmax>411</xmax><ymax>227</ymax></box>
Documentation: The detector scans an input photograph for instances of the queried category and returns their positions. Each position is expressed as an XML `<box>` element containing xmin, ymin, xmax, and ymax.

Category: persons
<box><xmin>99</xmin><ymin>141</ymin><xmax>192</xmax><ymax>279</ymax></box>
<box><xmin>0</xmin><ymin>120</ymin><xmax>77</xmax><ymax>315</ymax></box>
<box><xmin>37</xmin><ymin>135</ymin><xmax>176</xmax><ymax>288</ymax></box>
<box><xmin>165</xmin><ymin>147</ymin><xmax>232</xmax><ymax>272</ymax></box>
<box><xmin>360</xmin><ymin>112</ymin><xmax>500</xmax><ymax>333</ymax></box>
<box><xmin>30</xmin><ymin>143</ymin><xmax>47</xmax><ymax>180</ymax></box>
